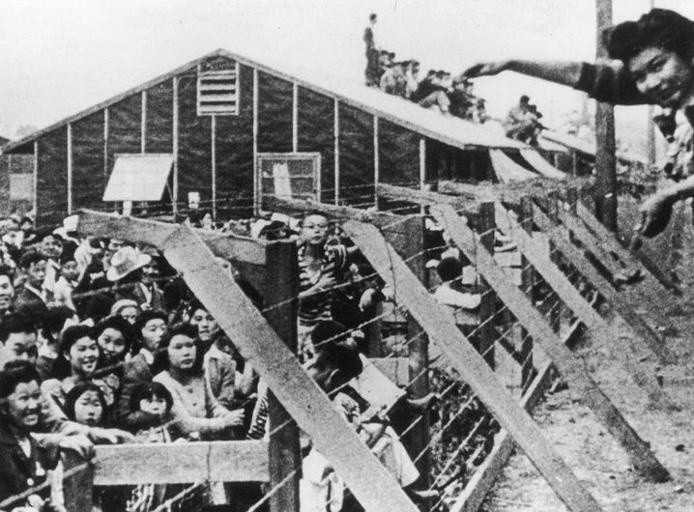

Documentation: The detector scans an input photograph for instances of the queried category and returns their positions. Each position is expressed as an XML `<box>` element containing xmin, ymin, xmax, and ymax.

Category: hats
<box><xmin>106</xmin><ymin>245</ymin><xmax>152</xmax><ymax>282</ymax></box>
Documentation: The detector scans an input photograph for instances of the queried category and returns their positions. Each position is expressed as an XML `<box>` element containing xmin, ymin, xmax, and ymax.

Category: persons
<box><xmin>363</xmin><ymin>11</ymin><xmax>486</xmax><ymax>123</ymax></box>
<box><xmin>459</xmin><ymin>9</ymin><xmax>693</xmax><ymax>238</ymax></box>
<box><xmin>0</xmin><ymin>208</ymin><xmax>419</xmax><ymax>511</ymax></box>
